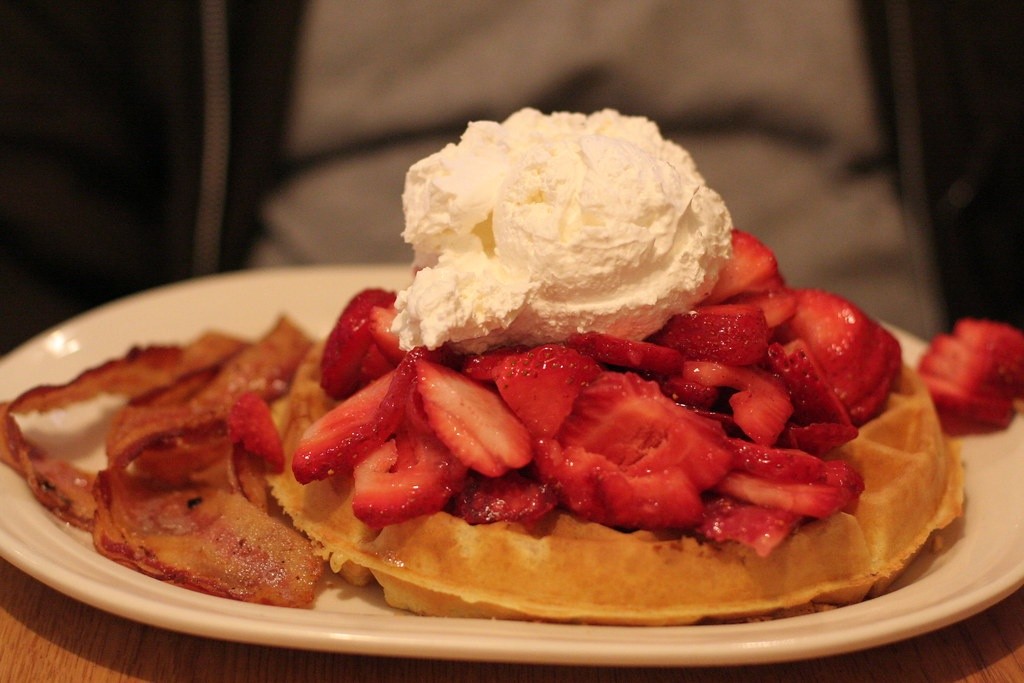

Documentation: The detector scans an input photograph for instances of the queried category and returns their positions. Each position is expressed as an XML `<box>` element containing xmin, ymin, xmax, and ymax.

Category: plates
<box><xmin>0</xmin><ymin>263</ymin><xmax>1024</xmax><ymax>670</ymax></box>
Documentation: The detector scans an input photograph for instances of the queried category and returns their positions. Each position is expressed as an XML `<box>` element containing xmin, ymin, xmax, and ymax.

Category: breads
<box><xmin>264</xmin><ymin>325</ymin><xmax>971</xmax><ymax>630</ymax></box>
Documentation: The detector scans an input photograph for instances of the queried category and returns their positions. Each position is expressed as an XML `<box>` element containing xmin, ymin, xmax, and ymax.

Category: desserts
<box><xmin>390</xmin><ymin>103</ymin><xmax>733</xmax><ymax>358</ymax></box>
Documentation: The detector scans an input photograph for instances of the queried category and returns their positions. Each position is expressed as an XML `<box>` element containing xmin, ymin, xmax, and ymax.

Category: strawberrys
<box><xmin>226</xmin><ymin>221</ymin><xmax>1023</xmax><ymax>556</ymax></box>
